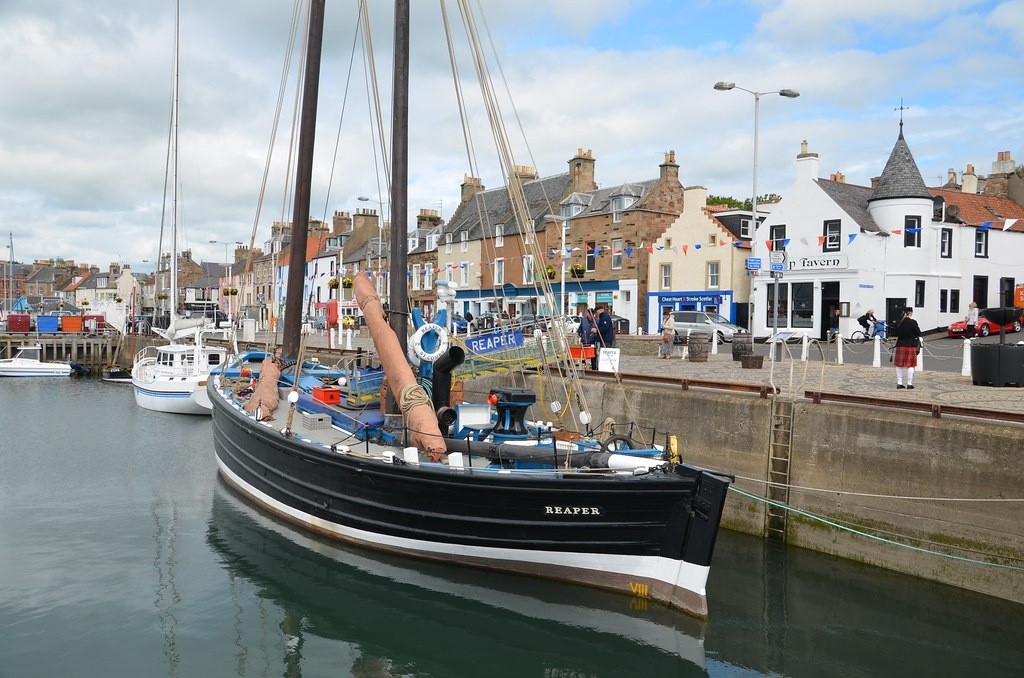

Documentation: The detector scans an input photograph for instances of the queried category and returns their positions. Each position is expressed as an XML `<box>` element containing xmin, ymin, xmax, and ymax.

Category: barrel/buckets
<box><xmin>741</xmin><ymin>355</ymin><xmax>763</xmax><ymax>369</ymax></box>
<box><xmin>688</xmin><ymin>332</ymin><xmax>710</xmax><ymax>362</ymax></box>
<box><xmin>732</xmin><ymin>333</ymin><xmax>753</xmax><ymax>361</ymax></box>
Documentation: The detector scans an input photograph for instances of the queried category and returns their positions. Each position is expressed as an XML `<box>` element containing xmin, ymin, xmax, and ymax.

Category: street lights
<box><xmin>713</xmin><ymin>80</ymin><xmax>800</xmax><ymax>350</ymax></box>
<box><xmin>210</xmin><ymin>240</ymin><xmax>242</xmax><ymax>284</ymax></box>
<box><xmin>357</xmin><ymin>197</ymin><xmax>390</xmax><ymax>300</ymax></box>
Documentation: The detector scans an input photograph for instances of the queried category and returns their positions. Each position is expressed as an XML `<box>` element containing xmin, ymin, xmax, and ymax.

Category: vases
<box><xmin>117</xmin><ymin>301</ymin><xmax>121</xmax><ymax>302</ymax></box>
<box><xmin>60</xmin><ymin>306</ymin><xmax>63</xmax><ymax>307</ymax></box>
<box><xmin>224</xmin><ymin>293</ymin><xmax>229</xmax><ymax>296</ymax></box>
<box><xmin>542</xmin><ymin>272</ymin><xmax>556</xmax><ymax>280</ymax></box>
<box><xmin>571</xmin><ymin>269</ymin><xmax>585</xmax><ymax>278</ymax></box>
<box><xmin>343</xmin><ymin>283</ymin><xmax>352</xmax><ymax>288</ymax></box>
<box><xmin>331</xmin><ymin>285</ymin><xmax>338</xmax><ymax>289</ymax></box>
<box><xmin>158</xmin><ymin>297</ymin><xmax>167</xmax><ymax>299</ymax></box>
<box><xmin>231</xmin><ymin>293</ymin><xmax>237</xmax><ymax>295</ymax></box>
<box><xmin>40</xmin><ymin>306</ymin><xmax>44</xmax><ymax>308</ymax></box>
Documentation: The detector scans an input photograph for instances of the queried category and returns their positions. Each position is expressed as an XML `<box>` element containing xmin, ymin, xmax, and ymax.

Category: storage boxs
<box><xmin>302</xmin><ymin>413</ymin><xmax>332</xmax><ymax>430</ymax></box>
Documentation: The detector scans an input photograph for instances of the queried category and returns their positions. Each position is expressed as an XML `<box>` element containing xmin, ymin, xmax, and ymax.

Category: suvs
<box><xmin>662</xmin><ymin>311</ymin><xmax>749</xmax><ymax>345</ymax></box>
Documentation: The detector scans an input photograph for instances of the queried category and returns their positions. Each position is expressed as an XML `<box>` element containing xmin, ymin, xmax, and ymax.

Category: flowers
<box><xmin>157</xmin><ymin>293</ymin><xmax>168</xmax><ymax>297</ymax></box>
<box><xmin>115</xmin><ymin>298</ymin><xmax>121</xmax><ymax>301</ymax></box>
<box><xmin>328</xmin><ymin>278</ymin><xmax>338</xmax><ymax>285</ymax></box>
<box><xmin>223</xmin><ymin>289</ymin><xmax>228</xmax><ymax>293</ymax></box>
<box><xmin>569</xmin><ymin>263</ymin><xmax>584</xmax><ymax>271</ymax></box>
<box><xmin>59</xmin><ymin>303</ymin><xmax>65</xmax><ymax>305</ymax></box>
<box><xmin>82</xmin><ymin>301</ymin><xmax>89</xmax><ymax>305</ymax></box>
<box><xmin>342</xmin><ymin>278</ymin><xmax>352</xmax><ymax>283</ymax></box>
<box><xmin>230</xmin><ymin>289</ymin><xmax>238</xmax><ymax>293</ymax></box>
<box><xmin>540</xmin><ymin>264</ymin><xmax>555</xmax><ymax>276</ymax></box>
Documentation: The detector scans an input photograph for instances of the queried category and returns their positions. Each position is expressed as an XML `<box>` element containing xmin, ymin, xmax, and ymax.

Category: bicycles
<box><xmin>851</xmin><ymin>320</ymin><xmax>888</xmax><ymax>345</ymax></box>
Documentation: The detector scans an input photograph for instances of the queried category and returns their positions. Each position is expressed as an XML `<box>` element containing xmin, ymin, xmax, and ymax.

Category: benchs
<box><xmin>658</xmin><ymin>335</ymin><xmax>689</xmax><ymax>359</ymax></box>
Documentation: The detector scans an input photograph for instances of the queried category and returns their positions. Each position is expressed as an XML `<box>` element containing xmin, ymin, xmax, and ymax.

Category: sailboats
<box><xmin>131</xmin><ymin>0</ymin><xmax>238</xmax><ymax>415</ymax></box>
<box><xmin>208</xmin><ymin>1</ymin><xmax>735</xmax><ymax>621</ymax></box>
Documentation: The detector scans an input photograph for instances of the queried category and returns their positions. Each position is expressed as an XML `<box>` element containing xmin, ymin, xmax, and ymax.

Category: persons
<box><xmin>962</xmin><ymin>302</ymin><xmax>979</xmax><ymax>339</ymax></box>
<box><xmin>661</xmin><ymin>312</ymin><xmax>675</xmax><ymax>358</ymax></box>
<box><xmin>466</xmin><ymin>310</ymin><xmax>473</xmax><ymax>322</ymax></box>
<box><xmin>829</xmin><ymin>308</ymin><xmax>840</xmax><ymax>340</ymax></box>
<box><xmin>859</xmin><ymin>309</ymin><xmax>876</xmax><ymax>336</ymax></box>
<box><xmin>894</xmin><ymin>307</ymin><xmax>921</xmax><ymax>389</ymax></box>
<box><xmin>592</xmin><ymin>306</ymin><xmax>614</xmax><ymax>348</ymax></box>
<box><xmin>579</xmin><ymin>308</ymin><xmax>599</xmax><ymax>370</ymax></box>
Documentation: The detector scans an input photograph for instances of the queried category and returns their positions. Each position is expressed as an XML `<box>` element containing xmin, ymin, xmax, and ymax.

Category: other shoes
<box><xmin>667</xmin><ymin>355</ymin><xmax>670</xmax><ymax>359</ymax></box>
<box><xmin>907</xmin><ymin>385</ymin><xmax>914</xmax><ymax>389</ymax></box>
<box><xmin>970</xmin><ymin>337</ymin><xmax>976</xmax><ymax>339</ymax></box>
<box><xmin>961</xmin><ymin>336</ymin><xmax>967</xmax><ymax>339</ymax></box>
<box><xmin>865</xmin><ymin>332</ymin><xmax>870</xmax><ymax>336</ymax></box>
<box><xmin>897</xmin><ymin>385</ymin><xmax>905</xmax><ymax>389</ymax></box>
<box><xmin>661</xmin><ymin>355</ymin><xmax>665</xmax><ymax>359</ymax></box>
<box><xmin>975</xmin><ymin>336</ymin><xmax>979</xmax><ymax>339</ymax></box>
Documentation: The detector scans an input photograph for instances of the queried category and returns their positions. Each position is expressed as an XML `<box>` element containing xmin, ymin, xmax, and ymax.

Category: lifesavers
<box><xmin>407</xmin><ymin>334</ymin><xmax>421</xmax><ymax>368</ymax></box>
<box><xmin>414</xmin><ymin>323</ymin><xmax>448</xmax><ymax>361</ymax></box>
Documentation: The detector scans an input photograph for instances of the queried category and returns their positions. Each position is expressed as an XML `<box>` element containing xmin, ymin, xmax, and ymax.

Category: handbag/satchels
<box><xmin>662</xmin><ymin>333</ymin><xmax>672</xmax><ymax>342</ymax></box>
<box><xmin>916</xmin><ymin>339</ymin><xmax>920</xmax><ymax>355</ymax></box>
<box><xmin>857</xmin><ymin>315</ymin><xmax>867</xmax><ymax>325</ymax></box>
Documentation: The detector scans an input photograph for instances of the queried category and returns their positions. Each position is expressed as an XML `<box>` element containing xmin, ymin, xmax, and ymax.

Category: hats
<box><xmin>594</xmin><ymin>306</ymin><xmax>603</xmax><ymax>311</ymax></box>
<box><xmin>903</xmin><ymin>307</ymin><xmax>912</xmax><ymax>312</ymax></box>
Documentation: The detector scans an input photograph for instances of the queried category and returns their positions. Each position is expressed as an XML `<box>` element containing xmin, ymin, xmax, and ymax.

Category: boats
<box><xmin>0</xmin><ymin>345</ymin><xmax>75</xmax><ymax>378</ymax></box>
<box><xmin>102</xmin><ymin>287</ymin><xmax>135</xmax><ymax>383</ymax></box>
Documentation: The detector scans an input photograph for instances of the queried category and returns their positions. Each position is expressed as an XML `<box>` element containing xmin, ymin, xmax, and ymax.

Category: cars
<box><xmin>947</xmin><ymin>308</ymin><xmax>1023</xmax><ymax>337</ymax></box>
<box><xmin>45</xmin><ymin>310</ymin><xmax>80</xmax><ymax>330</ymax></box>
<box><xmin>431</xmin><ymin>312</ymin><xmax>585</xmax><ymax>336</ymax></box>
<box><xmin>307</xmin><ymin>314</ymin><xmax>357</xmax><ymax>330</ymax></box>
